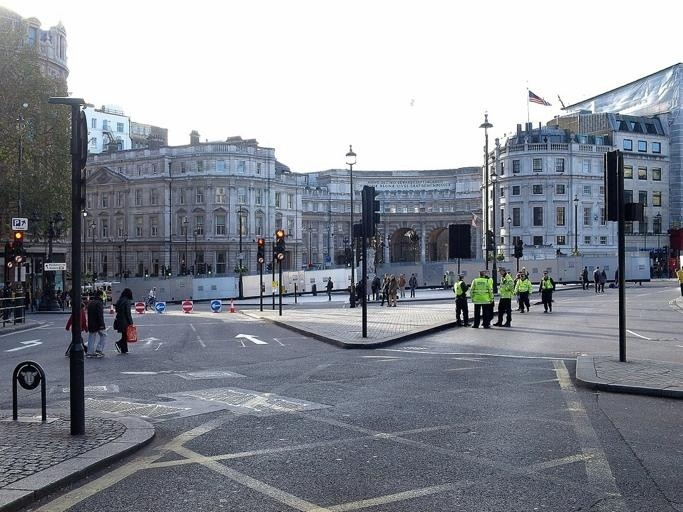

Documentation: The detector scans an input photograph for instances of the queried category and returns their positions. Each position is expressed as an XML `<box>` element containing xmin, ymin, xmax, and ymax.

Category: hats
<box><xmin>497</xmin><ymin>266</ymin><xmax>505</xmax><ymax>272</ymax></box>
<box><xmin>542</xmin><ymin>270</ymin><xmax>548</xmax><ymax>274</ymax></box>
<box><xmin>479</xmin><ymin>270</ymin><xmax>490</xmax><ymax>277</ymax></box>
<box><xmin>520</xmin><ymin>274</ymin><xmax>526</xmax><ymax>277</ymax></box>
<box><xmin>457</xmin><ymin>273</ymin><xmax>464</xmax><ymax>277</ymax></box>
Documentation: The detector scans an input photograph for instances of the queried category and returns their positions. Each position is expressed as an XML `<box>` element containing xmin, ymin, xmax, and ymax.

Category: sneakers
<box><xmin>482</xmin><ymin>325</ymin><xmax>490</xmax><ymax>328</ymax></box>
<box><xmin>515</xmin><ymin>308</ymin><xmax>553</xmax><ymax>313</ymax></box>
<box><xmin>501</xmin><ymin>322</ymin><xmax>510</xmax><ymax>327</ymax></box>
<box><xmin>493</xmin><ymin>321</ymin><xmax>502</xmax><ymax>326</ymax></box>
<box><xmin>463</xmin><ymin>323</ymin><xmax>471</xmax><ymax>327</ymax></box>
<box><xmin>471</xmin><ymin>325</ymin><xmax>478</xmax><ymax>328</ymax></box>
<box><xmin>114</xmin><ymin>343</ymin><xmax>120</xmax><ymax>353</ymax></box>
<box><xmin>64</xmin><ymin>350</ymin><xmax>104</xmax><ymax>358</ymax></box>
<box><xmin>456</xmin><ymin>323</ymin><xmax>464</xmax><ymax>326</ymax></box>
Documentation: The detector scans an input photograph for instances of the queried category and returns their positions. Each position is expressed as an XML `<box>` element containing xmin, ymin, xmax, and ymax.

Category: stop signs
<box><xmin>182</xmin><ymin>300</ymin><xmax>192</xmax><ymax>312</ymax></box>
<box><xmin>134</xmin><ymin>302</ymin><xmax>145</xmax><ymax>314</ymax></box>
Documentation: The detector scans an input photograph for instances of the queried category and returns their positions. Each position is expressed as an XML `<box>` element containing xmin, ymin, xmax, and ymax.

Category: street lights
<box><xmin>410</xmin><ymin>223</ymin><xmax>421</xmax><ymax>261</ymax></box>
<box><xmin>90</xmin><ymin>218</ymin><xmax>96</xmax><ymax>274</ymax></box>
<box><xmin>38</xmin><ymin>211</ymin><xmax>64</xmax><ymax>311</ymax></box>
<box><xmin>656</xmin><ymin>209</ymin><xmax>663</xmax><ymax>248</ymax></box>
<box><xmin>122</xmin><ymin>232</ymin><xmax>128</xmax><ymax>277</ymax></box>
<box><xmin>308</xmin><ymin>223</ymin><xmax>314</xmax><ymax>271</ymax></box>
<box><xmin>345</xmin><ymin>145</ymin><xmax>358</xmax><ymax>298</ymax></box>
<box><xmin>476</xmin><ymin>110</ymin><xmax>492</xmax><ymax>283</ymax></box>
<box><xmin>235</xmin><ymin>203</ymin><xmax>249</xmax><ymax>298</ymax></box>
<box><xmin>506</xmin><ymin>211</ymin><xmax>512</xmax><ymax>260</ymax></box>
<box><xmin>324</xmin><ymin>224</ymin><xmax>333</xmax><ymax>269</ymax></box>
<box><xmin>13</xmin><ymin>101</ymin><xmax>25</xmax><ymax>321</ymax></box>
<box><xmin>572</xmin><ymin>195</ymin><xmax>580</xmax><ymax>251</ymax></box>
<box><xmin>181</xmin><ymin>215</ymin><xmax>193</xmax><ymax>279</ymax></box>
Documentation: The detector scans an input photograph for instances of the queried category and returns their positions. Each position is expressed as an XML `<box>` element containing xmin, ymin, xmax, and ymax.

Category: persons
<box><xmin>324</xmin><ymin>277</ymin><xmax>333</xmax><ymax>301</ymax></box>
<box><xmin>676</xmin><ymin>266</ymin><xmax>683</xmax><ymax>296</ymax></box>
<box><xmin>1</xmin><ymin>287</ymin><xmax>156</xmax><ymax>358</ymax></box>
<box><xmin>453</xmin><ymin>266</ymin><xmax>556</xmax><ymax>328</ymax></box>
<box><xmin>354</xmin><ymin>272</ymin><xmax>418</xmax><ymax>307</ymax></box>
<box><xmin>443</xmin><ymin>270</ymin><xmax>449</xmax><ymax>289</ymax></box>
<box><xmin>581</xmin><ymin>263</ymin><xmax>618</xmax><ymax>293</ymax></box>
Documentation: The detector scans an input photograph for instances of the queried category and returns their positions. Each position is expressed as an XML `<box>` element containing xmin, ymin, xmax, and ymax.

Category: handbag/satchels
<box><xmin>126</xmin><ymin>325</ymin><xmax>137</xmax><ymax>342</ymax></box>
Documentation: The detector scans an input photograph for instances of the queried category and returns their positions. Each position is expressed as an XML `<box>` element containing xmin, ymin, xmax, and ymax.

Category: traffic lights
<box><xmin>258</xmin><ymin>239</ymin><xmax>264</xmax><ymax>258</ymax></box>
<box><xmin>276</xmin><ymin>230</ymin><xmax>285</xmax><ymax>254</ymax></box>
<box><xmin>373</xmin><ymin>190</ymin><xmax>383</xmax><ymax>225</ymax></box>
<box><xmin>516</xmin><ymin>240</ymin><xmax>523</xmax><ymax>258</ymax></box>
<box><xmin>14</xmin><ymin>232</ymin><xmax>22</xmax><ymax>255</ymax></box>
<box><xmin>487</xmin><ymin>230</ymin><xmax>495</xmax><ymax>251</ymax></box>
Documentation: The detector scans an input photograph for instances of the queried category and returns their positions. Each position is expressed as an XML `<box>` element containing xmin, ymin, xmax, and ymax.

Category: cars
<box><xmin>80</xmin><ymin>281</ymin><xmax>120</xmax><ymax>303</ymax></box>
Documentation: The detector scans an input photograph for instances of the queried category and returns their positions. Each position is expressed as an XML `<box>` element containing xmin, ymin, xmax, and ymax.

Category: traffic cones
<box><xmin>109</xmin><ymin>303</ymin><xmax>114</xmax><ymax>314</ymax></box>
<box><xmin>229</xmin><ymin>299</ymin><xmax>235</xmax><ymax>313</ymax></box>
